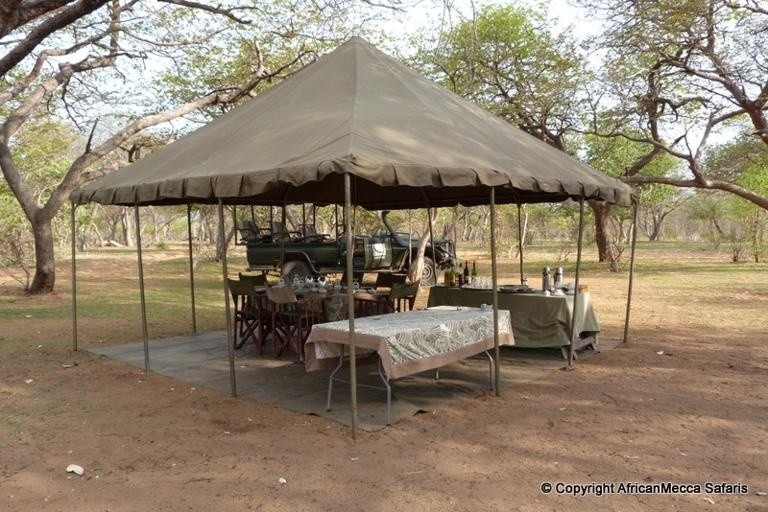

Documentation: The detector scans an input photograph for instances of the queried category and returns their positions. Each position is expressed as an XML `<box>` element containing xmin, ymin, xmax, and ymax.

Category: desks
<box><xmin>431</xmin><ymin>284</ymin><xmax>598</xmax><ymax>359</ymax></box>
<box><xmin>312</xmin><ymin>304</ymin><xmax>513</xmax><ymax>425</ymax></box>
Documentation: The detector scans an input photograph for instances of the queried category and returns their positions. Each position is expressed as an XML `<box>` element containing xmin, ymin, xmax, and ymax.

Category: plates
<box><xmin>501</xmin><ymin>284</ymin><xmax>531</xmax><ymax>294</ymax></box>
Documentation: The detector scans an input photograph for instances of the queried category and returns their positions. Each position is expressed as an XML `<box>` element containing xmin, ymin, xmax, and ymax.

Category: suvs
<box><xmin>233</xmin><ymin>203</ymin><xmax>458</xmax><ymax>286</ymax></box>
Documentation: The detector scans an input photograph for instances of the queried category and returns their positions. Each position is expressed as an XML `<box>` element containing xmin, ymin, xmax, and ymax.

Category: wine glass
<box><xmin>522</xmin><ymin>274</ymin><xmax>529</xmax><ymax>285</ymax></box>
<box><xmin>278</xmin><ymin>273</ymin><xmax>359</xmax><ymax>294</ymax></box>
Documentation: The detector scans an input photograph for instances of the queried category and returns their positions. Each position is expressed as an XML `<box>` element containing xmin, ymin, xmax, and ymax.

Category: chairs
<box><xmin>226</xmin><ymin>268</ymin><xmax>420</xmax><ymax>365</ymax></box>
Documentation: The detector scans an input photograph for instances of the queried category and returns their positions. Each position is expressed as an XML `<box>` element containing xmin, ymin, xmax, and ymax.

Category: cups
<box><xmin>470</xmin><ymin>275</ymin><xmax>495</xmax><ymax>288</ymax></box>
<box><xmin>542</xmin><ymin>267</ymin><xmax>563</xmax><ymax>292</ymax></box>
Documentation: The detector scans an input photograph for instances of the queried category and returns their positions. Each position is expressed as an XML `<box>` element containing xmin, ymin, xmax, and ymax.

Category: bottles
<box><xmin>444</xmin><ymin>261</ymin><xmax>477</xmax><ymax>287</ymax></box>
